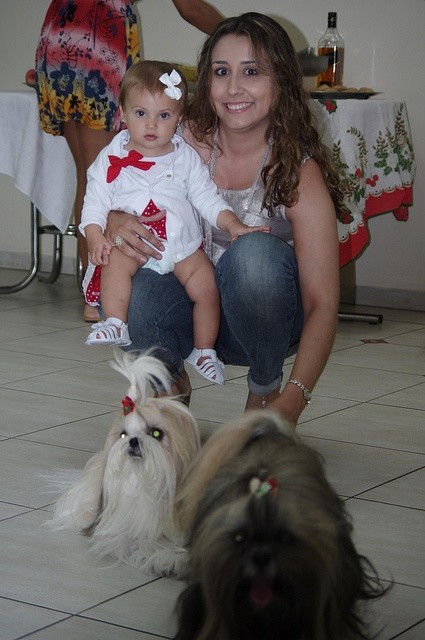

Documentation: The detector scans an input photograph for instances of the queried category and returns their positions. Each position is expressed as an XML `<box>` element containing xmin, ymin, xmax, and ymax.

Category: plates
<box><xmin>303</xmin><ymin>88</ymin><xmax>384</xmax><ymax>100</ymax></box>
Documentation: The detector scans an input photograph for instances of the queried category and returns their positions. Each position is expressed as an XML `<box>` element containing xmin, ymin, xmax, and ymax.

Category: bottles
<box><xmin>317</xmin><ymin>11</ymin><xmax>346</xmax><ymax>87</ymax></box>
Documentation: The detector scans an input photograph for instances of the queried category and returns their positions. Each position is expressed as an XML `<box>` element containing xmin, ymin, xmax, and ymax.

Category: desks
<box><xmin>0</xmin><ymin>89</ymin><xmax>412</xmax><ymax>324</ymax></box>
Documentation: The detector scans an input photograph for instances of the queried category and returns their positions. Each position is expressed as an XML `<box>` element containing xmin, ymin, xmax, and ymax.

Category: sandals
<box><xmin>185</xmin><ymin>348</ymin><xmax>224</xmax><ymax>385</ymax></box>
<box><xmin>85</xmin><ymin>318</ymin><xmax>131</xmax><ymax>347</ymax></box>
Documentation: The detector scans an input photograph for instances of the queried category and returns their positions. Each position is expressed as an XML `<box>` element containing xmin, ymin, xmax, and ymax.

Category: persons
<box><xmin>78</xmin><ymin>59</ymin><xmax>271</xmax><ymax>386</ymax></box>
<box><xmin>35</xmin><ymin>0</ymin><xmax>228</xmax><ymax>321</ymax></box>
<box><xmin>95</xmin><ymin>13</ymin><xmax>347</xmax><ymax>429</ymax></box>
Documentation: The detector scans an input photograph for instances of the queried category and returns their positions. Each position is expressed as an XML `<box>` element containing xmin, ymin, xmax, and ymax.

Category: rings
<box><xmin>113</xmin><ymin>236</ymin><xmax>123</xmax><ymax>247</ymax></box>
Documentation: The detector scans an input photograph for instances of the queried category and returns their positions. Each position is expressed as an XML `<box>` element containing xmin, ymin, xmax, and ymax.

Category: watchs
<box><xmin>286</xmin><ymin>379</ymin><xmax>311</xmax><ymax>404</ymax></box>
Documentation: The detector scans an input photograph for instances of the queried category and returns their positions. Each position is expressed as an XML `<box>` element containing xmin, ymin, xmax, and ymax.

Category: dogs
<box><xmin>42</xmin><ymin>346</ymin><xmax>201</xmax><ymax>580</ymax></box>
<box><xmin>166</xmin><ymin>409</ymin><xmax>396</xmax><ymax>640</ymax></box>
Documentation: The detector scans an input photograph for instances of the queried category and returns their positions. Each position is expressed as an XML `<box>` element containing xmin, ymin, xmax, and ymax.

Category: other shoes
<box><xmin>84</xmin><ymin>303</ymin><xmax>105</xmax><ymax>321</ymax></box>
<box><xmin>154</xmin><ymin>387</ymin><xmax>191</xmax><ymax>407</ymax></box>
